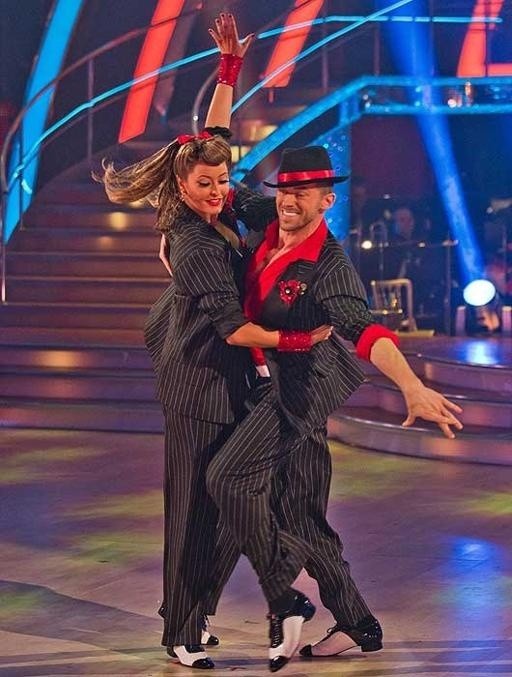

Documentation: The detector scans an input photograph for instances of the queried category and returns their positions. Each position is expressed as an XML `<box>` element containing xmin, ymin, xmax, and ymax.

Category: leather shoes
<box><xmin>267</xmin><ymin>591</ymin><xmax>316</xmax><ymax>673</ymax></box>
<box><xmin>158</xmin><ymin>607</ymin><xmax>218</xmax><ymax>645</ymax></box>
<box><xmin>167</xmin><ymin>644</ymin><xmax>214</xmax><ymax>669</ymax></box>
<box><xmin>299</xmin><ymin>619</ymin><xmax>383</xmax><ymax>658</ymax></box>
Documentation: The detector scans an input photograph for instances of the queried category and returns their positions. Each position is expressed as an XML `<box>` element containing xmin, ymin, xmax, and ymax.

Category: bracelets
<box><xmin>216</xmin><ymin>53</ymin><xmax>243</xmax><ymax>87</ymax></box>
<box><xmin>276</xmin><ymin>328</ymin><xmax>312</xmax><ymax>353</ymax></box>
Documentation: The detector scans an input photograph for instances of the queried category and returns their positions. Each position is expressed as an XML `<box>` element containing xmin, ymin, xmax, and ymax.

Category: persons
<box><xmin>91</xmin><ymin>12</ymin><xmax>334</xmax><ymax>668</ymax></box>
<box><xmin>204</xmin><ymin>145</ymin><xmax>463</xmax><ymax>672</ymax></box>
<box><xmin>364</xmin><ymin>192</ymin><xmax>512</xmax><ymax>334</ymax></box>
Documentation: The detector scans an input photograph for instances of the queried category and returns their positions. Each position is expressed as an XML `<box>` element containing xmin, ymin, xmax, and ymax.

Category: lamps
<box><xmin>462</xmin><ymin>276</ymin><xmax>505</xmax><ymax>338</ymax></box>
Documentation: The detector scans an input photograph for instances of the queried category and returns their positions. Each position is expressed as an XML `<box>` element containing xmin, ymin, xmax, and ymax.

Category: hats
<box><xmin>263</xmin><ymin>145</ymin><xmax>349</xmax><ymax>188</ymax></box>
